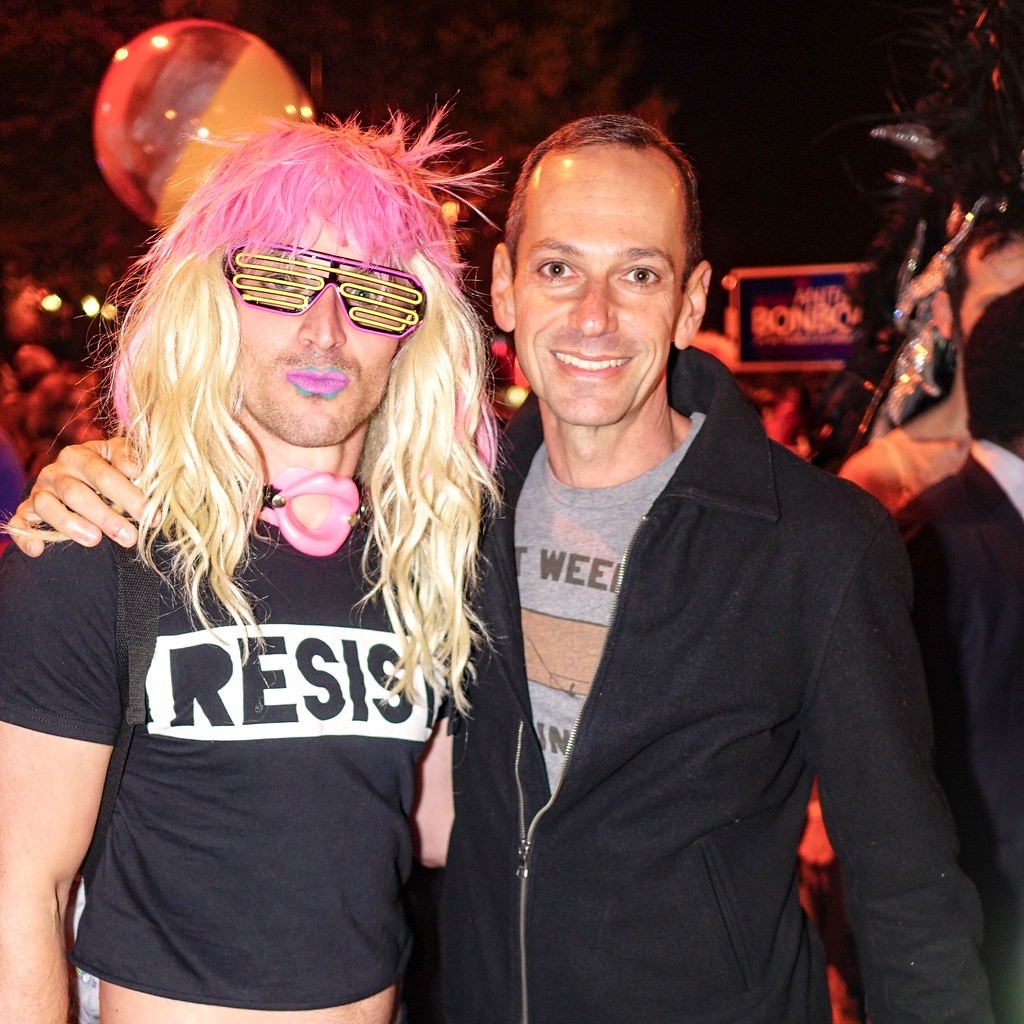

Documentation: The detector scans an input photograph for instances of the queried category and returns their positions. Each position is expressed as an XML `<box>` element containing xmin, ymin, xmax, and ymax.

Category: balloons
<box><xmin>96</xmin><ymin>22</ymin><xmax>315</xmax><ymax>229</ymax></box>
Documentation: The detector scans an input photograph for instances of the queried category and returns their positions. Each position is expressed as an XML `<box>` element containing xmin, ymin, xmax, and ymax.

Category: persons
<box><xmin>1</xmin><ymin>188</ymin><xmax>1023</xmax><ymax>1024</ymax></box>
<box><xmin>0</xmin><ymin>111</ymin><xmax>504</xmax><ymax>1024</ymax></box>
<box><xmin>0</xmin><ymin>117</ymin><xmax>994</xmax><ymax>1023</ymax></box>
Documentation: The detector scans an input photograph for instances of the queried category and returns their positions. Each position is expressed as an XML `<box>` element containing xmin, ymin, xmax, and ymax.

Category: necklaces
<box><xmin>235</xmin><ymin>471</ymin><xmax>364</xmax><ymax>560</ymax></box>
